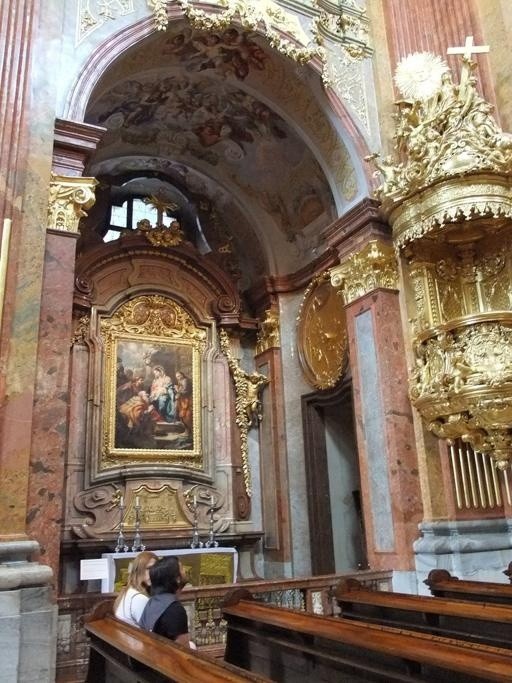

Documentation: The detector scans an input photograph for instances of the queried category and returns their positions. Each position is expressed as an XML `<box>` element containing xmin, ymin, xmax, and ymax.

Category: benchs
<box><xmin>77</xmin><ymin>561</ymin><xmax>512</xmax><ymax>683</ymax></box>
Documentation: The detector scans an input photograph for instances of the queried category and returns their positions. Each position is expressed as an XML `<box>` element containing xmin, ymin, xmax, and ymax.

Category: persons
<box><xmin>116</xmin><ymin>376</ymin><xmax>145</xmax><ymax>412</ymax></box>
<box><xmin>112</xmin><ymin>552</ymin><xmax>196</xmax><ymax>650</ymax></box>
<box><xmin>119</xmin><ymin>390</ymin><xmax>149</xmax><ymax>446</ymax></box>
<box><xmin>99</xmin><ymin>27</ymin><xmax>286</xmax><ymax>158</ymax></box>
<box><xmin>149</xmin><ymin>365</ymin><xmax>177</xmax><ymax>423</ymax></box>
<box><xmin>138</xmin><ymin>555</ymin><xmax>190</xmax><ymax>648</ymax></box>
<box><xmin>173</xmin><ymin>370</ymin><xmax>193</xmax><ymax>437</ymax></box>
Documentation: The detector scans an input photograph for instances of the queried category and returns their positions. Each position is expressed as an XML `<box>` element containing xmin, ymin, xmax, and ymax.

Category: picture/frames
<box><xmin>102</xmin><ymin>331</ymin><xmax>203</xmax><ymax>462</ymax></box>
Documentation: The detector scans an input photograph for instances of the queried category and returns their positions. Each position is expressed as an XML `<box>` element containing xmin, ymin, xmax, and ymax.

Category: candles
<box><xmin>135</xmin><ymin>495</ymin><xmax>140</xmax><ymax>506</ymax></box>
<box><xmin>211</xmin><ymin>496</ymin><xmax>214</xmax><ymax>504</ymax></box>
<box><xmin>193</xmin><ymin>496</ymin><xmax>198</xmax><ymax>504</ymax></box>
<box><xmin>119</xmin><ymin>494</ymin><xmax>124</xmax><ymax>505</ymax></box>
